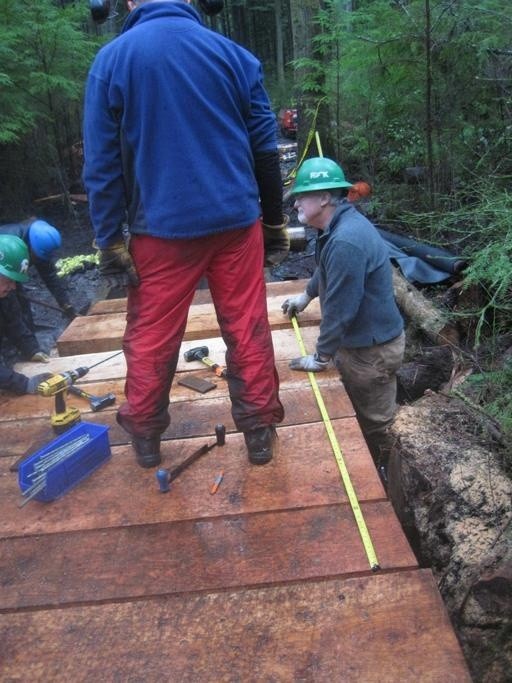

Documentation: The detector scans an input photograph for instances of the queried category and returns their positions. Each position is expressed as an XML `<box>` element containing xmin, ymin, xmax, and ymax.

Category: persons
<box><xmin>0</xmin><ymin>233</ymin><xmax>60</xmax><ymax>397</ymax></box>
<box><xmin>275</xmin><ymin>156</ymin><xmax>404</xmax><ymax>466</ymax></box>
<box><xmin>1</xmin><ymin>218</ymin><xmax>84</xmax><ymax>333</ymax></box>
<box><xmin>79</xmin><ymin>0</ymin><xmax>290</xmax><ymax>464</ymax></box>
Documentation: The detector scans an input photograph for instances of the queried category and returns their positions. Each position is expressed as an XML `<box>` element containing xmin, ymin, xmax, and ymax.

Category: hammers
<box><xmin>69</xmin><ymin>385</ymin><xmax>116</xmax><ymax>412</ymax></box>
<box><xmin>184</xmin><ymin>346</ymin><xmax>228</xmax><ymax>377</ymax></box>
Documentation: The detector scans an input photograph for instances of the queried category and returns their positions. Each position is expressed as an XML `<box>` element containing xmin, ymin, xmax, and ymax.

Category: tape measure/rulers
<box><xmin>290</xmin><ymin>309</ymin><xmax>381</xmax><ymax>572</ymax></box>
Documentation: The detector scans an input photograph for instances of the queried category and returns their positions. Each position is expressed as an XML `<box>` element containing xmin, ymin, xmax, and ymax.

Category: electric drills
<box><xmin>36</xmin><ymin>350</ymin><xmax>124</xmax><ymax>436</ymax></box>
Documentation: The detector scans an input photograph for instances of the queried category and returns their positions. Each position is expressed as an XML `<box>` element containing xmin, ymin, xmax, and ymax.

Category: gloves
<box><xmin>289</xmin><ymin>352</ymin><xmax>332</xmax><ymax>371</ymax></box>
<box><xmin>281</xmin><ymin>290</ymin><xmax>313</xmax><ymax>320</ymax></box>
<box><xmin>30</xmin><ymin>351</ymin><xmax>49</xmax><ymax>363</ymax></box>
<box><xmin>260</xmin><ymin>214</ymin><xmax>290</xmax><ymax>268</ymax></box>
<box><xmin>92</xmin><ymin>232</ymin><xmax>140</xmax><ymax>291</ymax></box>
<box><xmin>27</xmin><ymin>373</ymin><xmax>52</xmax><ymax>392</ymax></box>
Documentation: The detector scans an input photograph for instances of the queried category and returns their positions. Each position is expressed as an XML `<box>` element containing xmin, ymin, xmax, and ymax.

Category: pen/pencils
<box><xmin>210</xmin><ymin>470</ymin><xmax>224</xmax><ymax>495</ymax></box>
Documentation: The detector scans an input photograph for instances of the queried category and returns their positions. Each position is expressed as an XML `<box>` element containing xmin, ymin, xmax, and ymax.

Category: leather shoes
<box><xmin>242</xmin><ymin>427</ymin><xmax>273</xmax><ymax>465</ymax></box>
<box><xmin>134</xmin><ymin>436</ymin><xmax>161</xmax><ymax>468</ymax></box>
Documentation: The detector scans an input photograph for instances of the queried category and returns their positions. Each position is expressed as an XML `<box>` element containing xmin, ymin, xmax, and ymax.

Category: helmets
<box><xmin>28</xmin><ymin>220</ymin><xmax>61</xmax><ymax>258</ymax></box>
<box><xmin>1</xmin><ymin>235</ymin><xmax>30</xmax><ymax>283</ymax></box>
<box><xmin>288</xmin><ymin>157</ymin><xmax>352</xmax><ymax>194</ymax></box>
